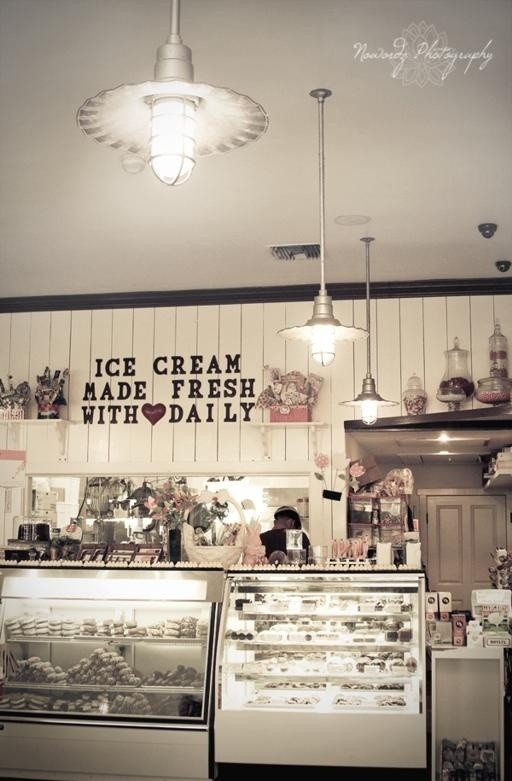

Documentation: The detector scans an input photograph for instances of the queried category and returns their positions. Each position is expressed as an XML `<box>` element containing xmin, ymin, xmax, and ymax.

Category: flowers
<box><xmin>315</xmin><ymin>454</ymin><xmax>364</xmax><ymax>492</ymax></box>
<box><xmin>149</xmin><ymin>481</ymin><xmax>196</xmax><ymax>529</ymax></box>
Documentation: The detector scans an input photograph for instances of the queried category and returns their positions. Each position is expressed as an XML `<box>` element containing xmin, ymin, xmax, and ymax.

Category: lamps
<box><xmin>338</xmin><ymin>238</ymin><xmax>398</xmax><ymax>426</ymax></box>
<box><xmin>279</xmin><ymin>89</ymin><xmax>368</xmax><ymax>367</ymax></box>
<box><xmin>78</xmin><ymin>0</ymin><xmax>269</xmax><ymax>186</ymax></box>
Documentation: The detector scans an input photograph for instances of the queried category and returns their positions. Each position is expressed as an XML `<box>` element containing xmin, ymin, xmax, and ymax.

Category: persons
<box><xmin>260</xmin><ymin>506</ymin><xmax>310</xmax><ymax>564</ymax></box>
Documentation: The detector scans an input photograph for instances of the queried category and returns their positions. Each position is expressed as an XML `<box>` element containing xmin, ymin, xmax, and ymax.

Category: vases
<box><xmin>169</xmin><ymin>529</ymin><xmax>181</xmax><ymax>562</ymax></box>
<box><xmin>323</xmin><ymin>489</ymin><xmax>342</xmax><ymax>501</ymax></box>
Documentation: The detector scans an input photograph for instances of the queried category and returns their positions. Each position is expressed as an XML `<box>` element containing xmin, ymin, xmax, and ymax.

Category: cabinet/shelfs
<box><xmin>432</xmin><ymin>647</ymin><xmax>505</xmax><ymax>780</ymax></box>
<box><xmin>0</xmin><ymin>560</ymin><xmax>223</xmax><ymax>781</ymax></box>
<box><xmin>213</xmin><ymin>567</ymin><xmax>426</xmax><ymax>781</ymax></box>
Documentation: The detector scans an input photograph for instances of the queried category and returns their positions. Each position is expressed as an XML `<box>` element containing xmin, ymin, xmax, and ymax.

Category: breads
<box><xmin>0</xmin><ymin>614</ymin><xmax>208</xmax><ymax>637</ymax></box>
<box><xmin>0</xmin><ymin>648</ymin><xmax>204</xmax><ymax>715</ymax></box>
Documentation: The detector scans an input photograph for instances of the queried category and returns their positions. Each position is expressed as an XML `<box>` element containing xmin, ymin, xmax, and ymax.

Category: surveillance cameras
<box><xmin>493</xmin><ymin>261</ymin><xmax>511</xmax><ymax>273</ymax></box>
<box><xmin>478</xmin><ymin>224</ymin><xmax>498</xmax><ymax>239</ymax></box>
